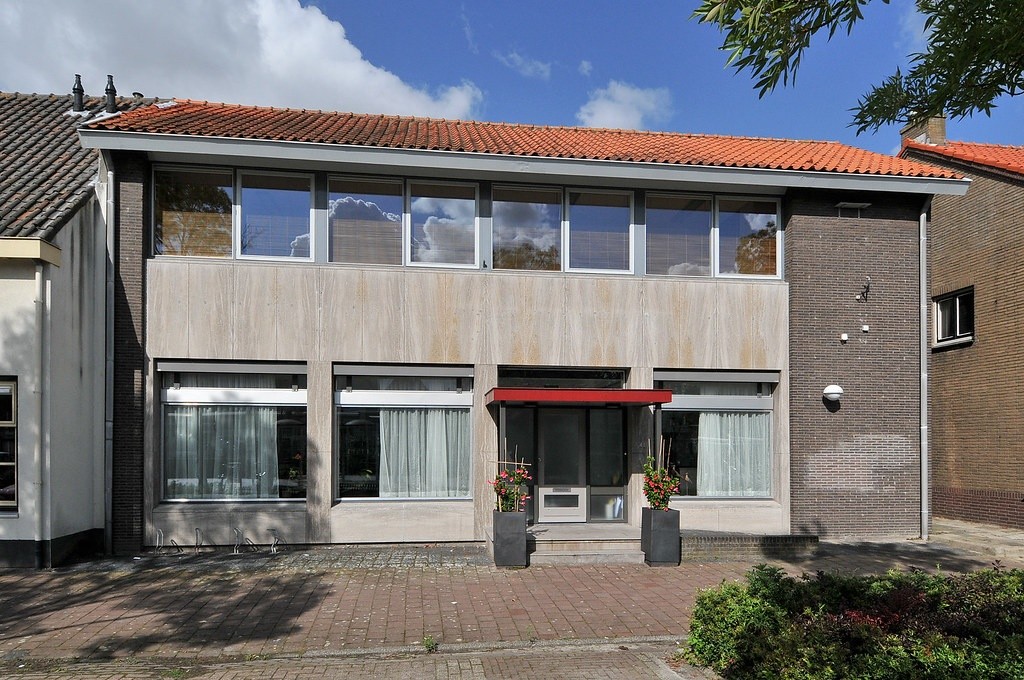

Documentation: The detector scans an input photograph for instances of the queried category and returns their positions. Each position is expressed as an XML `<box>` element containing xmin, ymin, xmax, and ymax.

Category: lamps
<box><xmin>822</xmin><ymin>384</ymin><xmax>845</xmax><ymax>401</ymax></box>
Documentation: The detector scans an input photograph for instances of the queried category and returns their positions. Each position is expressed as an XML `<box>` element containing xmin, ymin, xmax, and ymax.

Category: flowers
<box><xmin>486</xmin><ymin>466</ymin><xmax>532</xmax><ymax>511</ymax></box>
<box><xmin>641</xmin><ymin>453</ymin><xmax>683</xmax><ymax>511</ymax></box>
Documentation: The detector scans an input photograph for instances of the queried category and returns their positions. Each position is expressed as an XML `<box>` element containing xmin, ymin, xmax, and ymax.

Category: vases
<box><xmin>640</xmin><ymin>506</ymin><xmax>681</xmax><ymax>568</ymax></box>
<box><xmin>491</xmin><ymin>511</ymin><xmax>528</xmax><ymax>570</ymax></box>
